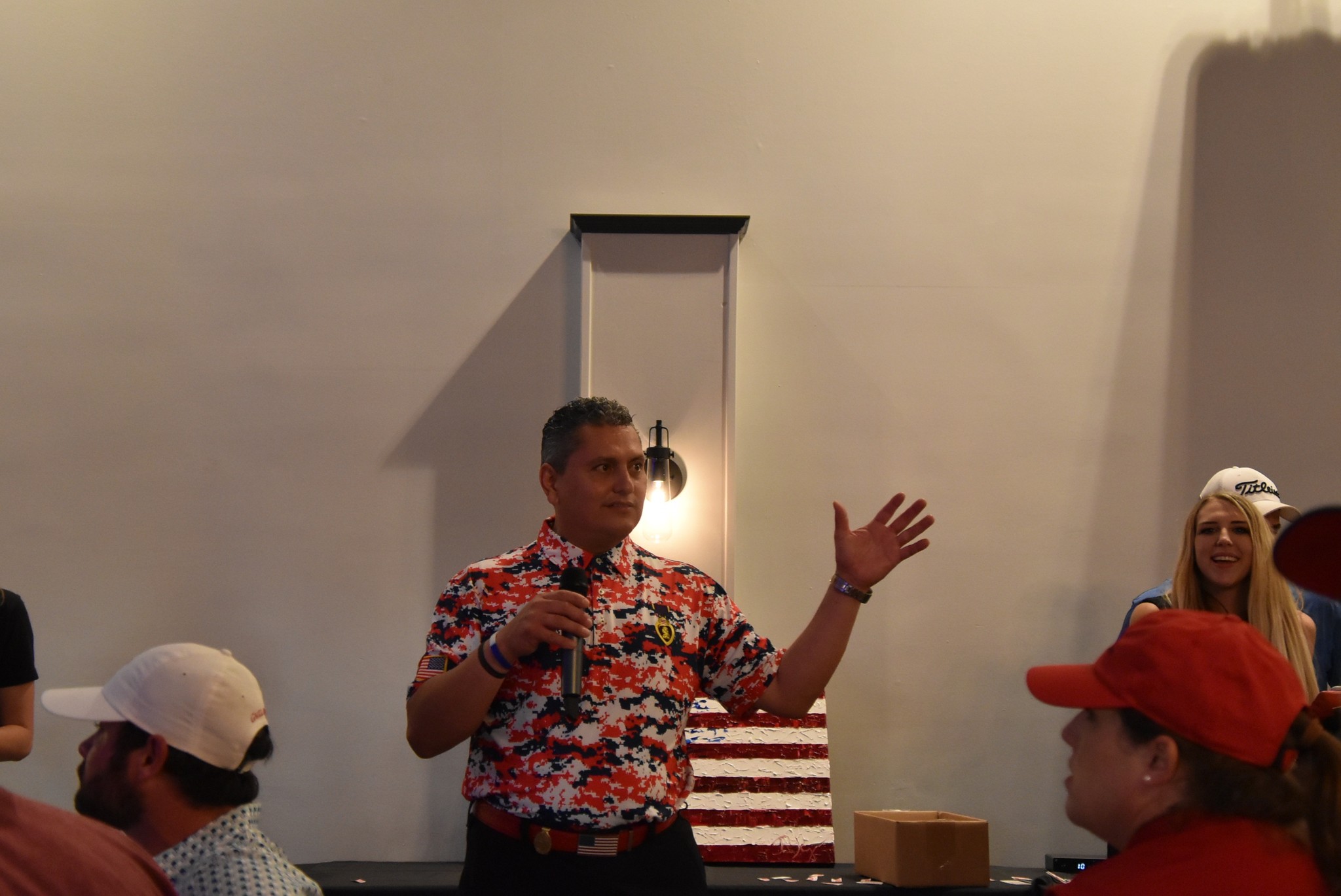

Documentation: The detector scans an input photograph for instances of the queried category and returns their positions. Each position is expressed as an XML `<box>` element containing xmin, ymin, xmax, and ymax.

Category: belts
<box><xmin>472</xmin><ymin>798</ymin><xmax>678</xmax><ymax>857</ymax></box>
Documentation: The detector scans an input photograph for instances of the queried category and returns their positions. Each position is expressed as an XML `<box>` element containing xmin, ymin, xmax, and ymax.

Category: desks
<box><xmin>297</xmin><ymin>860</ymin><xmax>1066</xmax><ymax>896</ymax></box>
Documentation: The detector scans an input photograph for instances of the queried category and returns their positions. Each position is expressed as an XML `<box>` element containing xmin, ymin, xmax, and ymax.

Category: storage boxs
<box><xmin>851</xmin><ymin>810</ymin><xmax>989</xmax><ymax>884</ymax></box>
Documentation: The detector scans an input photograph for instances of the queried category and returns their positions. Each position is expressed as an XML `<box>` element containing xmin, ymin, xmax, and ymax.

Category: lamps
<box><xmin>642</xmin><ymin>416</ymin><xmax>688</xmax><ymax>543</ymax></box>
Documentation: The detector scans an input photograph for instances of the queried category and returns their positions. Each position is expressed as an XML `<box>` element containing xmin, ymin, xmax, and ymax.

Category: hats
<box><xmin>1198</xmin><ymin>464</ymin><xmax>1302</xmax><ymax>526</ymax></box>
<box><xmin>1025</xmin><ymin>612</ymin><xmax>1306</xmax><ymax>768</ymax></box>
<box><xmin>40</xmin><ymin>642</ymin><xmax>271</xmax><ymax>769</ymax></box>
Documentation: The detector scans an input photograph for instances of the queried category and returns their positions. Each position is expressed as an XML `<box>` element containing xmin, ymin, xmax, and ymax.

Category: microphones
<box><xmin>558</xmin><ymin>567</ymin><xmax>587</xmax><ymax>721</ymax></box>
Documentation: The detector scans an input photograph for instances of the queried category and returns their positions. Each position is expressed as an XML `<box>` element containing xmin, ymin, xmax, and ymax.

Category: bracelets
<box><xmin>830</xmin><ymin>574</ymin><xmax>873</xmax><ymax>604</ymax></box>
<box><xmin>489</xmin><ymin>631</ymin><xmax>514</xmax><ymax>668</ymax></box>
<box><xmin>478</xmin><ymin>641</ymin><xmax>506</xmax><ymax>678</ymax></box>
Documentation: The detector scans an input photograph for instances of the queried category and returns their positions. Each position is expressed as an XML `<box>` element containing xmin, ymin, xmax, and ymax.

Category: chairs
<box><xmin>681</xmin><ymin>686</ymin><xmax>834</xmax><ymax>867</ymax></box>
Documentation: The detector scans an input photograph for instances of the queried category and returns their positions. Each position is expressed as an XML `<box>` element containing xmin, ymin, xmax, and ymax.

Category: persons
<box><xmin>0</xmin><ymin>587</ymin><xmax>39</xmax><ymax>762</ymax></box>
<box><xmin>1027</xmin><ymin>466</ymin><xmax>1341</xmax><ymax>895</ymax></box>
<box><xmin>0</xmin><ymin>786</ymin><xmax>181</xmax><ymax>896</ymax></box>
<box><xmin>408</xmin><ymin>398</ymin><xmax>934</xmax><ymax>896</ymax></box>
<box><xmin>40</xmin><ymin>642</ymin><xmax>323</xmax><ymax>896</ymax></box>
